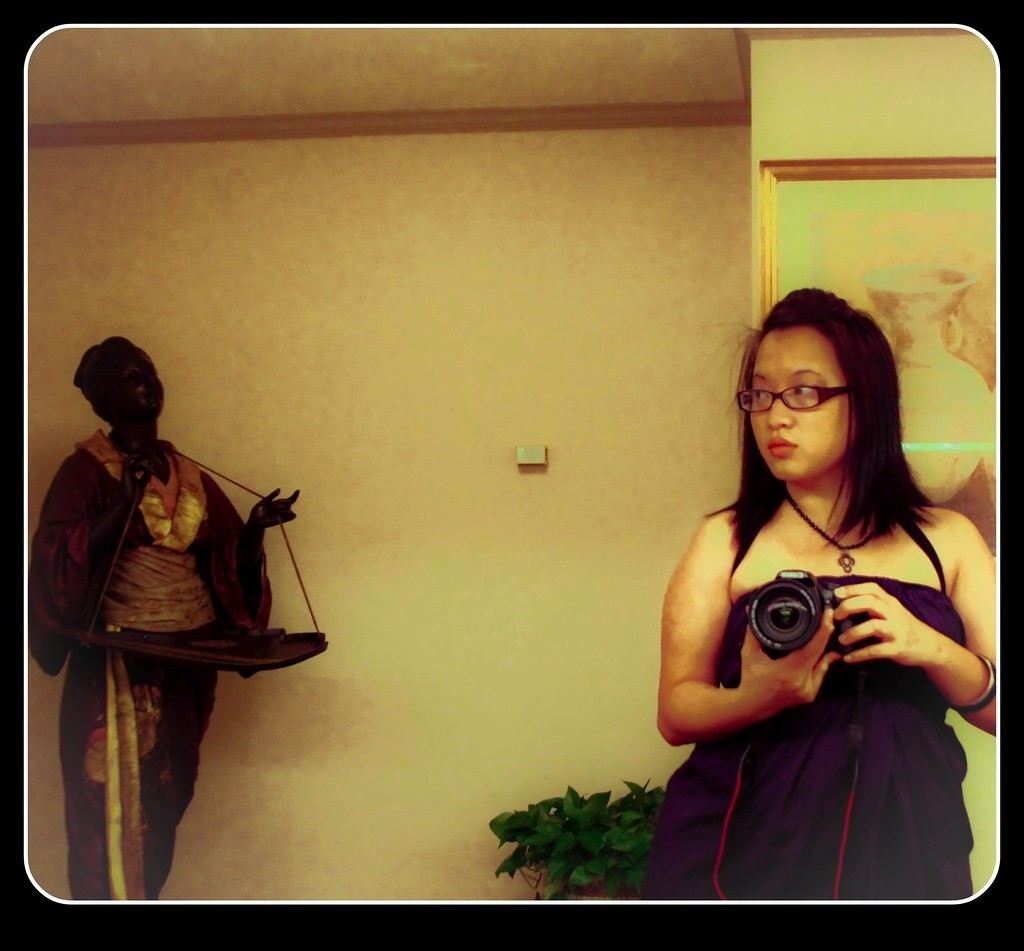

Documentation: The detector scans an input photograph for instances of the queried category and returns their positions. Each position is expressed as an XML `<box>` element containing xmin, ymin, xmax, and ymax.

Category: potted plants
<box><xmin>489</xmin><ymin>779</ymin><xmax>671</xmax><ymax>901</ymax></box>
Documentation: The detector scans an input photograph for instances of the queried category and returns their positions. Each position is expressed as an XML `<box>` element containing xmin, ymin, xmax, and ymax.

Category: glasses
<box><xmin>736</xmin><ymin>384</ymin><xmax>851</xmax><ymax>413</ymax></box>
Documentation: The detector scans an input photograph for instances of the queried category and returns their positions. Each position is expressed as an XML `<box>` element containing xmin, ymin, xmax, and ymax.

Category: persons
<box><xmin>27</xmin><ymin>338</ymin><xmax>299</xmax><ymax>902</ymax></box>
<box><xmin>645</xmin><ymin>289</ymin><xmax>998</xmax><ymax>900</ymax></box>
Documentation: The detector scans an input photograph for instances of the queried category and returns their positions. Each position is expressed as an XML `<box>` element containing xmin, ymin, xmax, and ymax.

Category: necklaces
<box><xmin>785</xmin><ymin>491</ymin><xmax>875</xmax><ymax>572</ymax></box>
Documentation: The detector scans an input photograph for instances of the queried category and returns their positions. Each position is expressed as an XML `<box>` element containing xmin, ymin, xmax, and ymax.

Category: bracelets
<box><xmin>951</xmin><ymin>652</ymin><xmax>996</xmax><ymax>712</ymax></box>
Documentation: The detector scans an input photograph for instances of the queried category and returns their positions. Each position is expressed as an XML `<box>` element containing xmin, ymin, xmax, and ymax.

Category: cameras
<box><xmin>746</xmin><ymin>570</ymin><xmax>853</xmax><ymax>671</ymax></box>
